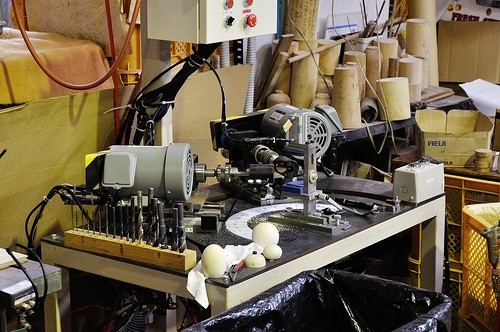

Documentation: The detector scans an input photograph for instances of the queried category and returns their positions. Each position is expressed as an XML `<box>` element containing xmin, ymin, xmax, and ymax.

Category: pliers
<box><xmin>227</xmin><ymin>259</ymin><xmax>245</xmax><ymax>282</ymax></box>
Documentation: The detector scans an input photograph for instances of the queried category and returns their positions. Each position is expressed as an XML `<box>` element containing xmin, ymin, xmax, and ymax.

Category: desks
<box><xmin>39</xmin><ymin>173</ymin><xmax>446</xmax><ymax>332</ymax></box>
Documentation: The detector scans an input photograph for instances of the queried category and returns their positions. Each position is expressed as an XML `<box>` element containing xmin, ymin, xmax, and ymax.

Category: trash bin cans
<box><xmin>179</xmin><ymin>269</ymin><xmax>451</xmax><ymax>332</ymax></box>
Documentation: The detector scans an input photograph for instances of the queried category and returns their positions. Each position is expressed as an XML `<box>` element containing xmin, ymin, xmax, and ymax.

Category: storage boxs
<box><xmin>415</xmin><ymin>109</ymin><xmax>494</xmax><ymax>167</ymax></box>
<box><xmin>458</xmin><ymin>203</ymin><xmax>500</xmax><ymax>332</ymax></box>
